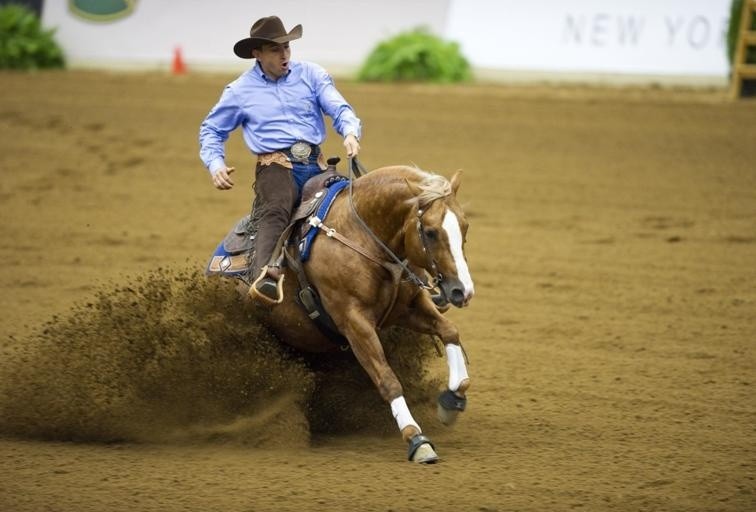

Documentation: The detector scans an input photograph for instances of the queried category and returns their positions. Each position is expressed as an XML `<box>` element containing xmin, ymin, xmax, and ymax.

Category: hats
<box><xmin>234</xmin><ymin>16</ymin><xmax>304</xmax><ymax>59</ymax></box>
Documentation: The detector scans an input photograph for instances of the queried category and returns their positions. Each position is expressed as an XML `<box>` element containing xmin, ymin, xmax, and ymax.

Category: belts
<box><xmin>284</xmin><ymin>141</ymin><xmax>320</xmax><ymax>160</ymax></box>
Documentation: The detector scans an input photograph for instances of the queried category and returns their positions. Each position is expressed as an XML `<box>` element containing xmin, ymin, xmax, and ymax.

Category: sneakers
<box><xmin>257</xmin><ymin>282</ymin><xmax>279</xmax><ymax>300</ymax></box>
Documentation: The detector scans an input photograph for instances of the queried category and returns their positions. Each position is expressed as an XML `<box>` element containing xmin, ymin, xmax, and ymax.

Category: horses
<box><xmin>205</xmin><ymin>164</ymin><xmax>476</xmax><ymax>464</ymax></box>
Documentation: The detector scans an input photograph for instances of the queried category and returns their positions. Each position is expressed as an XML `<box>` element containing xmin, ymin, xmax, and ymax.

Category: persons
<box><xmin>196</xmin><ymin>15</ymin><xmax>363</xmax><ymax>312</ymax></box>
